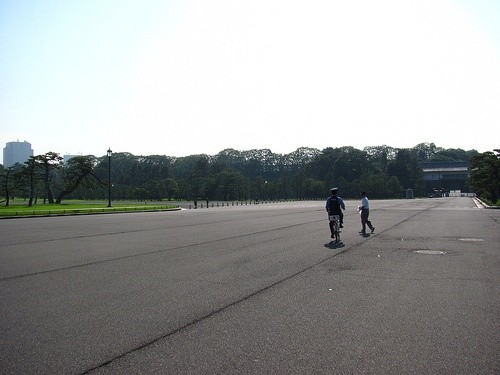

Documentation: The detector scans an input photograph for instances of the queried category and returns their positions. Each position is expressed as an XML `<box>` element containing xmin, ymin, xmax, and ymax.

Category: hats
<box><xmin>330</xmin><ymin>188</ymin><xmax>338</xmax><ymax>193</ymax></box>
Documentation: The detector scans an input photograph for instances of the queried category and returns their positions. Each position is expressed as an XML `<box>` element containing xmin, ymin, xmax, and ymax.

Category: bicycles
<box><xmin>330</xmin><ymin>215</ymin><xmax>343</xmax><ymax>243</ymax></box>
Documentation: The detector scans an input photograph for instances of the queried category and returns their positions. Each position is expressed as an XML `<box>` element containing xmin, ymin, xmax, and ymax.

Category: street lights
<box><xmin>107</xmin><ymin>146</ymin><xmax>112</xmax><ymax>207</ymax></box>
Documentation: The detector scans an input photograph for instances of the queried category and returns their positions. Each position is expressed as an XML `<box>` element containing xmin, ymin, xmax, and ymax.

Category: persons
<box><xmin>358</xmin><ymin>192</ymin><xmax>375</xmax><ymax>233</ymax></box>
<box><xmin>325</xmin><ymin>187</ymin><xmax>345</xmax><ymax>238</ymax></box>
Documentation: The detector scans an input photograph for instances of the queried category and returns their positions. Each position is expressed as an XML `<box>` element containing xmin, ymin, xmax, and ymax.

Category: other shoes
<box><xmin>371</xmin><ymin>228</ymin><xmax>374</xmax><ymax>233</ymax></box>
<box><xmin>359</xmin><ymin>232</ymin><xmax>365</xmax><ymax>234</ymax></box>
<box><xmin>331</xmin><ymin>234</ymin><xmax>335</xmax><ymax>238</ymax></box>
<box><xmin>340</xmin><ymin>225</ymin><xmax>343</xmax><ymax>228</ymax></box>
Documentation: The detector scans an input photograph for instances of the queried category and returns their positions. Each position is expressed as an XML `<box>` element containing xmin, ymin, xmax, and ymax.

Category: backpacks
<box><xmin>330</xmin><ymin>196</ymin><xmax>339</xmax><ymax>212</ymax></box>
